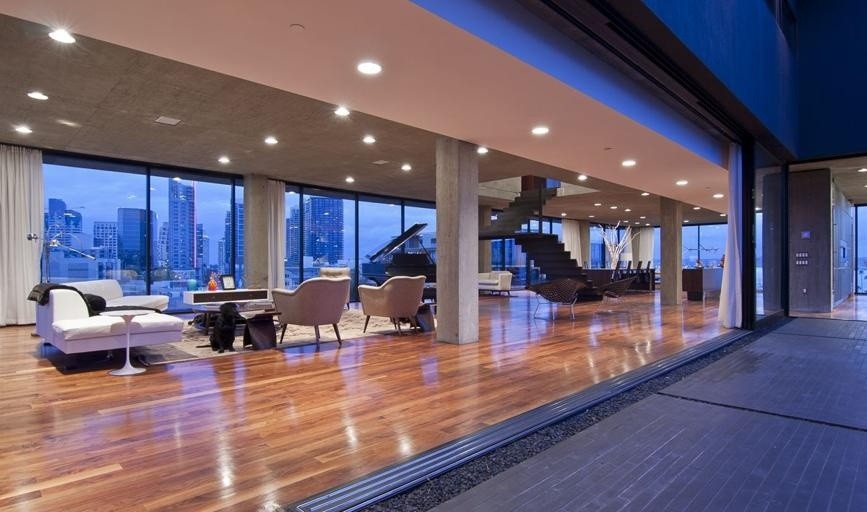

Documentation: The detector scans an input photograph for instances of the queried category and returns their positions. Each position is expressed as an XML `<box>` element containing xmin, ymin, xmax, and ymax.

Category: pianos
<box><xmin>362</xmin><ymin>226</ymin><xmax>436</xmax><ymax>328</ymax></box>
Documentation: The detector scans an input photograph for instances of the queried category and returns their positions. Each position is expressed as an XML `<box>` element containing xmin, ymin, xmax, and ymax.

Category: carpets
<box><xmin>132</xmin><ymin>308</ymin><xmax>434</xmax><ymax>367</ymax></box>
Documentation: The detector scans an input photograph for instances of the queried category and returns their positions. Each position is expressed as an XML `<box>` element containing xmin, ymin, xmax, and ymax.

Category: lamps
<box><xmin>27</xmin><ymin>233</ymin><xmax>60</xmax><ymax>338</ymax></box>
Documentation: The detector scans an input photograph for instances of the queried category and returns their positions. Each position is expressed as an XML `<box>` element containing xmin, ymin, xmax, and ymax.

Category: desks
<box><xmin>182</xmin><ymin>288</ymin><xmax>269</xmax><ymax>328</ymax></box>
<box><xmin>681</xmin><ymin>267</ymin><xmax>722</xmax><ymax>301</ymax></box>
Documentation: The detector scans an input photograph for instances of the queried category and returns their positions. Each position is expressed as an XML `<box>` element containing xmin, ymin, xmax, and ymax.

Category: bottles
<box><xmin>208</xmin><ymin>274</ymin><xmax>217</xmax><ymax>291</ymax></box>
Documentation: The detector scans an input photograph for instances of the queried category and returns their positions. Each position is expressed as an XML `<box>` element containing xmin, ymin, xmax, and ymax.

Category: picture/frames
<box><xmin>220</xmin><ymin>274</ymin><xmax>236</xmax><ymax>290</ymax></box>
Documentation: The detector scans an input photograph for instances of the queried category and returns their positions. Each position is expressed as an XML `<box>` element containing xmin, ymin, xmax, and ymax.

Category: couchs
<box><xmin>35</xmin><ymin>279</ymin><xmax>184</xmax><ymax>370</ymax></box>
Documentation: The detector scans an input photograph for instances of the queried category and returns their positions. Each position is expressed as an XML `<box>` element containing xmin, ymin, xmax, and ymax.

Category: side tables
<box><xmin>95</xmin><ymin>310</ymin><xmax>154</xmax><ymax>376</ymax></box>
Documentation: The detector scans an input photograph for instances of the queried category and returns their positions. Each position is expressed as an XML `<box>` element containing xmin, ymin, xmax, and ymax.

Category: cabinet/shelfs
<box><xmin>786</xmin><ymin>168</ymin><xmax>855</xmax><ymax>314</ymax></box>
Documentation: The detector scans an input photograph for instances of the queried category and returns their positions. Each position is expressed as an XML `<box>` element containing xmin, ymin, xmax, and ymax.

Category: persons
<box><xmin>719</xmin><ymin>254</ymin><xmax>725</xmax><ymax>268</ymax></box>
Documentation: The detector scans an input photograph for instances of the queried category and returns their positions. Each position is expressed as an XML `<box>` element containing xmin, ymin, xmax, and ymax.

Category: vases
<box><xmin>209</xmin><ymin>274</ymin><xmax>217</xmax><ymax>290</ymax></box>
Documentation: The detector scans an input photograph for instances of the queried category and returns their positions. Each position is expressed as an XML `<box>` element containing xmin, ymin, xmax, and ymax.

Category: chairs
<box><xmin>525</xmin><ymin>275</ymin><xmax>639</xmax><ymax>320</ymax></box>
<box><xmin>272</xmin><ymin>267</ymin><xmax>427</xmax><ymax>349</ymax></box>
<box><xmin>477</xmin><ymin>271</ymin><xmax>512</xmax><ymax>297</ymax></box>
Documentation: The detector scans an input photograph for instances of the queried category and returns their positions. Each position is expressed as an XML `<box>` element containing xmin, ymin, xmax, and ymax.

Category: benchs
<box><xmin>192</xmin><ymin>304</ymin><xmax>282</xmax><ymax>349</ymax></box>
